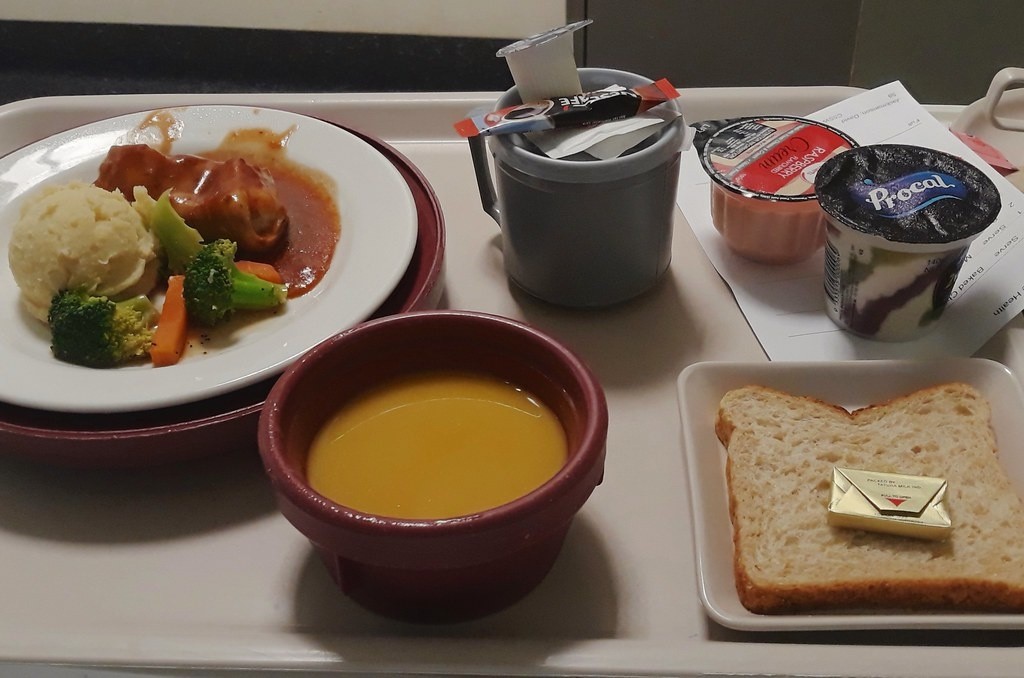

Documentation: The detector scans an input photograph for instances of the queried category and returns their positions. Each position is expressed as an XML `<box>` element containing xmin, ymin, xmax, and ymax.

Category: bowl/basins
<box><xmin>259</xmin><ymin>310</ymin><xmax>609</xmax><ymax>627</ymax></box>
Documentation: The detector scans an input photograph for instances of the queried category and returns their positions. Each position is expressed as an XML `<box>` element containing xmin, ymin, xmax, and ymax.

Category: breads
<box><xmin>715</xmin><ymin>382</ymin><xmax>1024</xmax><ymax>614</ymax></box>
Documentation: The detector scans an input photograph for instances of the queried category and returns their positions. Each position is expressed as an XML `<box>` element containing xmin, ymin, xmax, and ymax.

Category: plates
<box><xmin>0</xmin><ymin>108</ymin><xmax>448</xmax><ymax>471</ymax></box>
<box><xmin>950</xmin><ymin>88</ymin><xmax>1024</xmax><ymax>194</ymax></box>
<box><xmin>0</xmin><ymin>104</ymin><xmax>419</xmax><ymax>413</ymax></box>
<box><xmin>676</xmin><ymin>353</ymin><xmax>1024</xmax><ymax>633</ymax></box>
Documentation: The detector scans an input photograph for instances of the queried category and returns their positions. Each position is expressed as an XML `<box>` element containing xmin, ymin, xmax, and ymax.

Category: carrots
<box><xmin>234</xmin><ymin>260</ymin><xmax>282</xmax><ymax>284</ymax></box>
<box><xmin>149</xmin><ymin>274</ymin><xmax>189</xmax><ymax>365</ymax></box>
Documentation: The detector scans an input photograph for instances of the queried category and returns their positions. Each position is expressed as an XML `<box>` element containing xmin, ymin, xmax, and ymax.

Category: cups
<box><xmin>812</xmin><ymin>144</ymin><xmax>999</xmax><ymax>343</ymax></box>
<box><xmin>703</xmin><ymin>117</ymin><xmax>858</xmax><ymax>265</ymax></box>
<box><xmin>465</xmin><ymin>66</ymin><xmax>686</xmax><ymax>311</ymax></box>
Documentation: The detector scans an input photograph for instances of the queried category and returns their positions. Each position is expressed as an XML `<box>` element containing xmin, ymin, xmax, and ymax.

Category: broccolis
<box><xmin>47</xmin><ymin>189</ymin><xmax>290</xmax><ymax>369</ymax></box>
